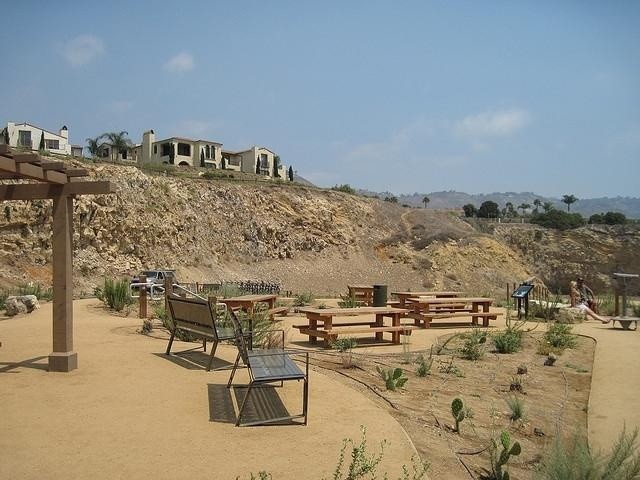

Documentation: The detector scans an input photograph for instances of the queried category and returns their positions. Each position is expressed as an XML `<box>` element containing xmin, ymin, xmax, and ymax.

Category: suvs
<box><xmin>130</xmin><ymin>271</ymin><xmax>177</xmax><ymax>294</ymax></box>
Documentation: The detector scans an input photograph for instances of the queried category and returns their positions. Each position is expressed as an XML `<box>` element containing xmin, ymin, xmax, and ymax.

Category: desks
<box><xmin>347</xmin><ymin>283</ymin><xmax>374</xmax><ymax>308</ymax></box>
<box><xmin>217</xmin><ymin>294</ymin><xmax>278</xmax><ymax>327</ymax></box>
<box><xmin>612</xmin><ymin>273</ymin><xmax>639</xmax><ymax>316</ymax></box>
<box><xmin>294</xmin><ymin>307</ymin><xmax>415</xmax><ymax>350</ymax></box>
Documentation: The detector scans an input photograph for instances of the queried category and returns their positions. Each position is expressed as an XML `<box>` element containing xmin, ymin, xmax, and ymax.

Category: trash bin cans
<box><xmin>373</xmin><ymin>285</ymin><xmax>388</xmax><ymax>307</ymax></box>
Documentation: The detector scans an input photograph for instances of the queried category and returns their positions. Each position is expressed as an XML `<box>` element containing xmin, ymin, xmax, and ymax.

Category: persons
<box><xmin>575</xmin><ymin>278</ymin><xmax>596</xmax><ymax>321</ymax></box>
<box><xmin>567</xmin><ymin>281</ymin><xmax>610</xmax><ymax>324</ymax></box>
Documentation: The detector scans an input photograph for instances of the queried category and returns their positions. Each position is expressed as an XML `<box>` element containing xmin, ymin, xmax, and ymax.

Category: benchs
<box><xmin>317</xmin><ymin>326</ymin><xmax>419</xmax><ymax>340</ymax></box>
<box><xmin>255</xmin><ymin>307</ymin><xmax>289</xmax><ymax>321</ymax></box>
<box><xmin>166</xmin><ymin>294</ymin><xmax>252</xmax><ymax>372</ymax></box>
<box><xmin>361</xmin><ymin>294</ymin><xmax>373</xmax><ymax>307</ymax></box>
<box><xmin>340</xmin><ymin>293</ymin><xmax>361</xmax><ymax>308</ymax></box>
<box><xmin>391</xmin><ymin>291</ymin><xmax>503</xmax><ymax>328</ymax></box>
<box><xmin>226</xmin><ymin>302</ymin><xmax>308</xmax><ymax>427</ymax></box>
<box><xmin>292</xmin><ymin>322</ymin><xmax>386</xmax><ymax>337</ymax></box>
